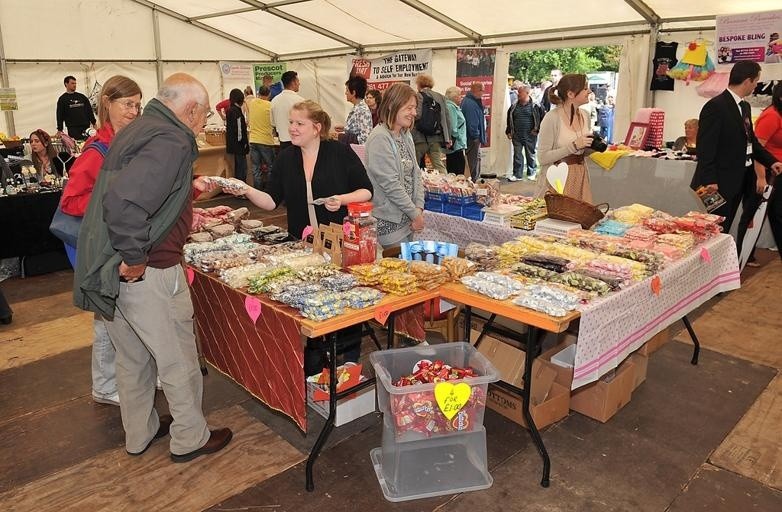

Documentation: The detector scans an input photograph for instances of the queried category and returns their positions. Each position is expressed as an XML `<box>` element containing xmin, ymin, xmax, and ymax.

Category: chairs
<box><xmin>393</xmin><ymin>239</ymin><xmax>461</xmax><ymax>348</ymax></box>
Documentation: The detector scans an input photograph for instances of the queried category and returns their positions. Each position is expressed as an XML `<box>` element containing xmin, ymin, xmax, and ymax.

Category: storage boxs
<box><xmin>305</xmin><ymin>363</ymin><xmax>376</xmax><ymax>428</ymax></box>
<box><xmin>469</xmin><ymin>327</ymin><xmax>558</xmax><ymax>405</ymax></box>
<box><xmin>369</xmin><ymin>414</ymin><xmax>494</xmax><ymax>503</ymax></box>
<box><xmin>538</xmin><ymin>341</ymin><xmax>635</xmax><ymax>424</ymax></box>
<box><xmin>486</xmin><ymin>382</ymin><xmax>571</xmax><ymax>431</ymax></box>
<box><xmin>369</xmin><ymin>339</ymin><xmax>501</xmax><ymax>442</ymax></box>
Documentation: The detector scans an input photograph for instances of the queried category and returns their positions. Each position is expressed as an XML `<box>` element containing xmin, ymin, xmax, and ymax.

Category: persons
<box><xmin>222</xmin><ymin>100</ymin><xmax>373</xmax><ymax>375</ymax></box>
<box><xmin>226</xmin><ymin>88</ymin><xmax>250</xmax><ymax>199</ymax></box>
<box><xmin>445</xmin><ymin>86</ymin><xmax>467</xmax><ymax>174</ymax></box>
<box><xmin>216</xmin><ymin>98</ymin><xmax>230</xmax><ymax>121</ymax></box>
<box><xmin>718</xmin><ymin>47</ymin><xmax>729</xmax><ymax>62</ymax></box>
<box><xmin>242</xmin><ymin>86</ymin><xmax>256</xmax><ymax>126</ymax></box>
<box><xmin>737</xmin><ymin>80</ymin><xmax>782</xmax><ymax>264</ymax></box>
<box><xmin>364</xmin><ymin>83</ymin><xmax>425</xmax><ymax>248</ymax></box>
<box><xmin>672</xmin><ymin>118</ymin><xmax>698</xmax><ymax>155</ymax></box>
<box><xmin>72</xmin><ymin>72</ymin><xmax>233</xmax><ymax>465</ymax></box>
<box><xmin>249</xmin><ymin>85</ymin><xmax>276</xmax><ymax>190</ymax></box>
<box><xmin>534</xmin><ymin>74</ymin><xmax>604</xmax><ymax>205</ymax></box>
<box><xmin>270</xmin><ymin>71</ymin><xmax>307</xmax><ymax>206</ymax></box>
<box><xmin>505</xmin><ymin>85</ymin><xmax>546</xmax><ymax>182</ymax></box>
<box><xmin>410</xmin><ymin>75</ymin><xmax>452</xmax><ymax>174</ymax></box>
<box><xmin>601</xmin><ymin>96</ymin><xmax>615</xmax><ymax>108</ymax></box>
<box><xmin>49</xmin><ymin>76</ymin><xmax>217</xmax><ymax>408</ymax></box>
<box><xmin>511</xmin><ymin>80</ymin><xmax>540</xmax><ymax>107</ymax></box>
<box><xmin>21</xmin><ymin>130</ymin><xmax>58</xmax><ymax>182</ymax></box>
<box><xmin>457</xmin><ymin>50</ymin><xmax>495</xmax><ymax>76</ymax></box>
<box><xmin>366</xmin><ymin>90</ymin><xmax>382</xmax><ymax>126</ymax></box>
<box><xmin>334</xmin><ymin>76</ymin><xmax>372</xmax><ymax>167</ymax></box>
<box><xmin>690</xmin><ymin>60</ymin><xmax>782</xmax><ymax>266</ymax></box>
<box><xmin>541</xmin><ymin>69</ymin><xmax>563</xmax><ymax>111</ymax></box>
<box><xmin>56</xmin><ymin>76</ymin><xmax>98</xmax><ymax>139</ymax></box>
<box><xmin>460</xmin><ymin>82</ymin><xmax>487</xmax><ymax>182</ymax></box>
<box><xmin>769</xmin><ymin>33</ymin><xmax>781</xmax><ymax>54</ymax></box>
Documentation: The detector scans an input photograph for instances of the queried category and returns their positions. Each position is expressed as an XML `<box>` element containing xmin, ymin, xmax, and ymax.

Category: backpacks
<box><xmin>415</xmin><ymin>85</ymin><xmax>449</xmax><ymax>150</ymax></box>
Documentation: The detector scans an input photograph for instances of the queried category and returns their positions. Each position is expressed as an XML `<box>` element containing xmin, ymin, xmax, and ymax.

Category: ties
<box><xmin>739</xmin><ymin>99</ymin><xmax>750</xmax><ymax>133</ymax></box>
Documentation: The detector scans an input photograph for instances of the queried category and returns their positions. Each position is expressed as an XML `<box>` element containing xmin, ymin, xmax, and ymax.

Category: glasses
<box><xmin>197</xmin><ymin>102</ymin><xmax>215</xmax><ymax>120</ymax></box>
<box><xmin>111</xmin><ymin>99</ymin><xmax>143</xmax><ymax>113</ymax></box>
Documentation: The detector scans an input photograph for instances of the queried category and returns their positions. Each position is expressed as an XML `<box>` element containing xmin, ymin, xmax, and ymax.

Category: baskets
<box><xmin>544</xmin><ymin>190</ymin><xmax>609</xmax><ymax>230</ymax></box>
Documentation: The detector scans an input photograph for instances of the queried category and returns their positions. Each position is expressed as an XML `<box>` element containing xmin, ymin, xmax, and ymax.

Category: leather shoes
<box><xmin>171</xmin><ymin>427</ymin><xmax>232</xmax><ymax>463</ymax></box>
<box><xmin>125</xmin><ymin>420</ymin><xmax>169</xmax><ymax>457</ymax></box>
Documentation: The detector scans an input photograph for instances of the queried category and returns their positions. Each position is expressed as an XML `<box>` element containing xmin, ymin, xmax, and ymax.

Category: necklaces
<box><xmin>56</xmin><ymin>154</ymin><xmax>72</xmax><ymax>175</ymax></box>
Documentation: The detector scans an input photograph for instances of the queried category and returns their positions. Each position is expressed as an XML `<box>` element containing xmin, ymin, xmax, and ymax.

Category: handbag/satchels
<box><xmin>48</xmin><ymin>141</ymin><xmax>108</xmax><ymax>251</ymax></box>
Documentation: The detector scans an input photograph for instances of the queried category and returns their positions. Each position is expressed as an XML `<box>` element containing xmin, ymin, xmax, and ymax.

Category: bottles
<box><xmin>342</xmin><ymin>202</ymin><xmax>377</xmax><ymax>272</ymax></box>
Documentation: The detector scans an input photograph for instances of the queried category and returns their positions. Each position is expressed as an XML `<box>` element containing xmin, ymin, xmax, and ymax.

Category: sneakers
<box><xmin>527</xmin><ymin>173</ymin><xmax>537</xmax><ymax>180</ymax></box>
<box><xmin>508</xmin><ymin>175</ymin><xmax>522</xmax><ymax>181</ymax></box>
<box><xmin>91</xmin><ymin>388</ymin><xmax>120</xmax><ymax>406</ymax></box>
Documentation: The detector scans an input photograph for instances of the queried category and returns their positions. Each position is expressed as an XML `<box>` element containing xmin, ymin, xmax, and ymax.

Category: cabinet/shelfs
<box><xmin>440</xmin><ymin>213</ymin><xmax>743</xmax><ymax>488</ymax></box>
<box><xmin>182</xmin><ymin>205</ymin><xmax>440</xmax><ymax>492</ymax></box>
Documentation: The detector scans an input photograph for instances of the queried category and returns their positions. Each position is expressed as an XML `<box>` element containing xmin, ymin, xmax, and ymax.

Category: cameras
<box><xmin>587</xmin><ymin>135</ymin><xmax>607</xmax><ymax>153</ymax></box>
